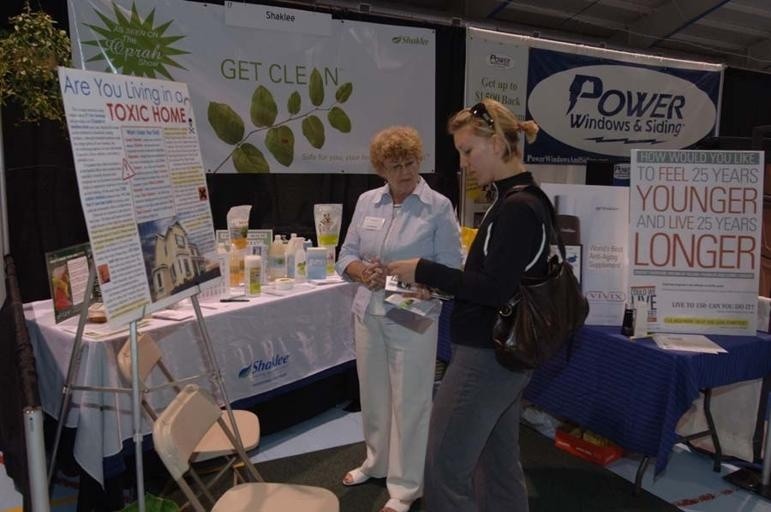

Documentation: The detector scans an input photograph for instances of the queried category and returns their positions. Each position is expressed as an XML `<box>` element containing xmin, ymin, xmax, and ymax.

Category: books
<box><xmin>652</xmin><ymin>333</ymin><xmax>728</xmax><ymax>355</ymax></box>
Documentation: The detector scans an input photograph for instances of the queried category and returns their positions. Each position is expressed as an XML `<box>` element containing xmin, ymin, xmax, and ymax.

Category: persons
<box><xmin>388</xmin><ymin>99</ymin><xmax>567</xmax><ymax>512</ymax></box>
<box><xmin>336</xmin><ymin>126</ymin><xmax>463</xmax><ymax>512</ymax></box>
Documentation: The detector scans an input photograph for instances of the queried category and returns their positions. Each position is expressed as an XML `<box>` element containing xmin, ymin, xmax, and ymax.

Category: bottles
<box><xmin>269</xmin><ymin>233</ymin><xmax>306</xmax><ymax>278</ymax></box>
<box><xmin>216</xmin><ymin>243</ymin><xmax>262</xmax><ymax>297</ymax></box>
<box><xmin>622</xmin><ymin>300</ymin><xmax>650</xmax><ymax>338</ymax></box>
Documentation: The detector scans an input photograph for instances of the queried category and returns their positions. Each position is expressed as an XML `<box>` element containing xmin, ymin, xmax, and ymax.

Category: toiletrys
<box><xmin>620</xmin><ymin>302</ymin><xmax>633</xmax><ymax>337</ymax></box>
<box><xmin>305</xmin><ymin>247</ymin><xmax>327</xmax><ymax>279</ymax></box>
<box><xmin>245</xmin><ymin>255</ymin><xmax>262</xmax><ymax>295</ymax></box>
<box><xmin>633</xmin><ymin>302</ymin><xmax>649</xmax><ymax>336</ymax></box>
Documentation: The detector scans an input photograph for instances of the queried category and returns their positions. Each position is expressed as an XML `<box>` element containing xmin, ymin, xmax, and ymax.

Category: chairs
<box><xmin>115</xmin><ymin>331</ymin><xmax>261</xmax><ymax>508</ymax></box>
<box><xmin>152</xmin><ymin>384</ymin><xmax>340</xmax><ymax>512</ymax></box>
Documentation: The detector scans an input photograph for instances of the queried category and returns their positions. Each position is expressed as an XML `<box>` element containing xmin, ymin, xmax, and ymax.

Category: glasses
<box><xmin>469</xmin><ymin>102</ymin><xmax>494</xmax><ymax>130</ymax></box>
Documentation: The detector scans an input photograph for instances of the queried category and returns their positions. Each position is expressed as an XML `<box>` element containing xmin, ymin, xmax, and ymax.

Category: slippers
<box><xmin>379</xmin><ymin>499</ymin><xmax>415</xmax><ymax>512</ymax></box>
<box><xmin>342</xmin><ymin>466</ymin><xmax>371</xmax><ymax>485</ymax></box>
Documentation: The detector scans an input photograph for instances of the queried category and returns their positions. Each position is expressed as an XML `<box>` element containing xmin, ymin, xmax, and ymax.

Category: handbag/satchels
<box><xmin>491</xmin><ymin>183</ymin><xmax>589</xmax><ymax>374</ymax></box>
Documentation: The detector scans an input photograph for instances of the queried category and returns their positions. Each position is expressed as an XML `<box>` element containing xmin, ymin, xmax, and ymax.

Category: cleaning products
<box><xmin>266</xmin><ymin>234</ymin><xmax>287</xmax><ymax>279</ymax></box>
<box><xmin>291</xmin><ymin>237</ymin><xmax>305</xmax><ymax>278</ymax></box>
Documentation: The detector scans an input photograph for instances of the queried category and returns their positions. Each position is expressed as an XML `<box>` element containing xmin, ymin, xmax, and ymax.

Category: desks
<box><xmin>15</xmin><ymin>272</ymin><xmax>404</xmax><ymax>512</ymax></box>
<box><xmin>436</xmin><ymin>294</ymin><xmax>771</xmax><ymax>504</ymax></box>
